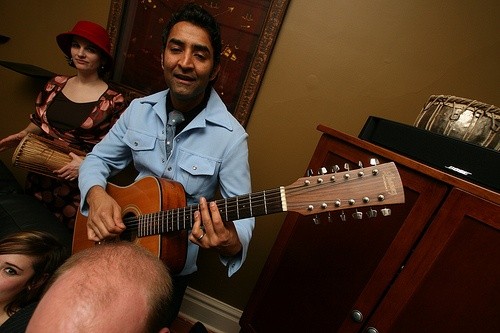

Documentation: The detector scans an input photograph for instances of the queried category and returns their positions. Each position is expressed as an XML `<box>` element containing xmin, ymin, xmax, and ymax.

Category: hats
<box><xmin>55</xmin><ymin>20</ymin><xmax>113</xmax><ymax>61</ymax></box>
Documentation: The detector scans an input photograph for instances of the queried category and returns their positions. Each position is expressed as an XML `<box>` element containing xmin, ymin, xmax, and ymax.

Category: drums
<box><xmin>414</xmin><ymin>94</ymin><xmax>500</xmax><ymax>151</ymax></box>
<box><xmin>12</xmin><ymin>133</ymin><xmax>88</xmax><ymax>182</ymax></box>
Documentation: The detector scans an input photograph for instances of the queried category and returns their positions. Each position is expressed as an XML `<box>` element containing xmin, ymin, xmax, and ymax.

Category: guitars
<box><xmin>71</xmin><ymin>158</ymin><xmax>406</xmax><ymax>273</ymax></box>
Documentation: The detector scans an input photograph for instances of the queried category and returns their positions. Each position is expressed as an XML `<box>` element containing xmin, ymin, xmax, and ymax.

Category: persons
<box><xmin>26</xmin><ymin>237</ymin><xmax>173</xmax><ymax>333</ymax></box>
<box><xmin>0</xmin><ymin>20</ymin><xmax>128</xmax><ymax>232</ymax></box>
<box><xmin>78</xmin><ymin>3</ymin><xmax>256</xmax><ymax>323</ymax></box>
<box><xmin>0</xmin><ymin>231</ymin><xmax>66</xmax><ymax>333</ymax></box>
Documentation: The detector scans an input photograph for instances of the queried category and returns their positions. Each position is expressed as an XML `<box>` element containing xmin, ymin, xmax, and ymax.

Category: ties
<box><xmin>167</xmin><ymin>111</ymin><xmax>184</xmax><ymax>161</ymax></box>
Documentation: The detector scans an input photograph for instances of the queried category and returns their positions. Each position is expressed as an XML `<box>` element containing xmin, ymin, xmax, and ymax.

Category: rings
<box><xmin>197</xmin><ymin>234</ymin><xmax>204</xmax><ymax>240</ymax></box>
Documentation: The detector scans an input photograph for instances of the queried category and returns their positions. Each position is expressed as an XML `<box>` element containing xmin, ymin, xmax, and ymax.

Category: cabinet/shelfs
<box><xmin>240</xmin><ymin>124</ymin><xmax>499</xmax><ymax>332</ymax></box>
